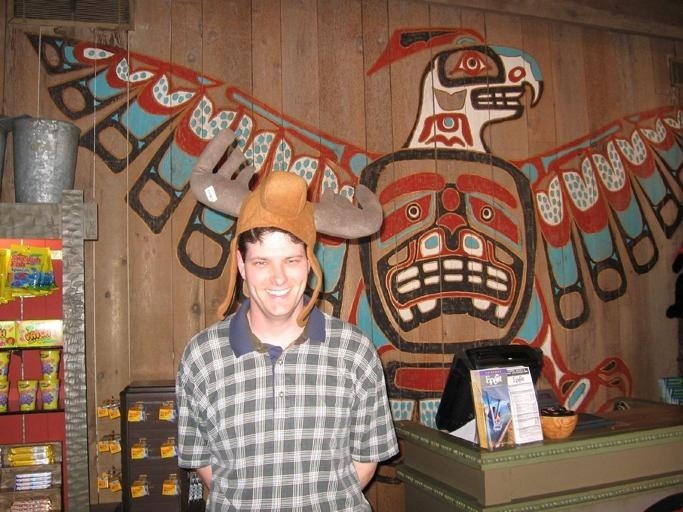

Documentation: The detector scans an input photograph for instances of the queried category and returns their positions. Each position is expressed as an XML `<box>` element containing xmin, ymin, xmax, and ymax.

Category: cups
<box><xmin>0</xmin><ymin>349</ymin><xmax>59</xmax><ymax>413</ymax></box>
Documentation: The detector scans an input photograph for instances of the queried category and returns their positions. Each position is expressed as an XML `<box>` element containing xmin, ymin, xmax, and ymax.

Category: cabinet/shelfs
<box><xmin>119</xmin><ymin>380</ymin><xmax>188</xmax><ymax>512</ymax></box>
<box><xmin>0</xmin><ymin>189</ymin><xmax>98</xmax><ymax>512</ymax></box>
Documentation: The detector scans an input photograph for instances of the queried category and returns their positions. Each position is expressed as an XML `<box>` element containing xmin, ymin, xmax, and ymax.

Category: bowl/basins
<box><xmin>538</xmin><ymin>411</ymin><xmax>579</xmax><ymax>440</ymax></box>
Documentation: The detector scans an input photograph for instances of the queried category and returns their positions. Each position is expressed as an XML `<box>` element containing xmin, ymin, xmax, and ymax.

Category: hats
<box><xmin>232</xmin><ymin>169</ymin><xmax>317</xmax><ymax>250</ymax></box>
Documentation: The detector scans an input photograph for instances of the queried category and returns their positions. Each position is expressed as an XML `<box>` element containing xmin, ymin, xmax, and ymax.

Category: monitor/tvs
<box><xmin>435</xmin><ymin>345</ymin><xmax>544</xmax><ymax>433</ymax></box>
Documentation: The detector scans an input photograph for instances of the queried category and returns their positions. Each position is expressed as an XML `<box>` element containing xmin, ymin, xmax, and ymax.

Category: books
<box><xmin>470</xmin><ymin>364</ymin><xmax>544</xmax><ymax>450</ymax></box>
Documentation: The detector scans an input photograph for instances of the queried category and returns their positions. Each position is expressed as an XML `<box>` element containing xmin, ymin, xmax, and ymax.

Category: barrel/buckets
<box><xmin>13</xmin><ymin>117</ymin><xmax>79</xmax><ymax>203</ymax></box>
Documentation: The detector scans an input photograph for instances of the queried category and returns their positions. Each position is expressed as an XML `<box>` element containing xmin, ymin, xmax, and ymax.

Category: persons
<box><xmin>174</xmin><ymin>227</ymin><xmax>401</xmax><ymax>512</ymax></box>
